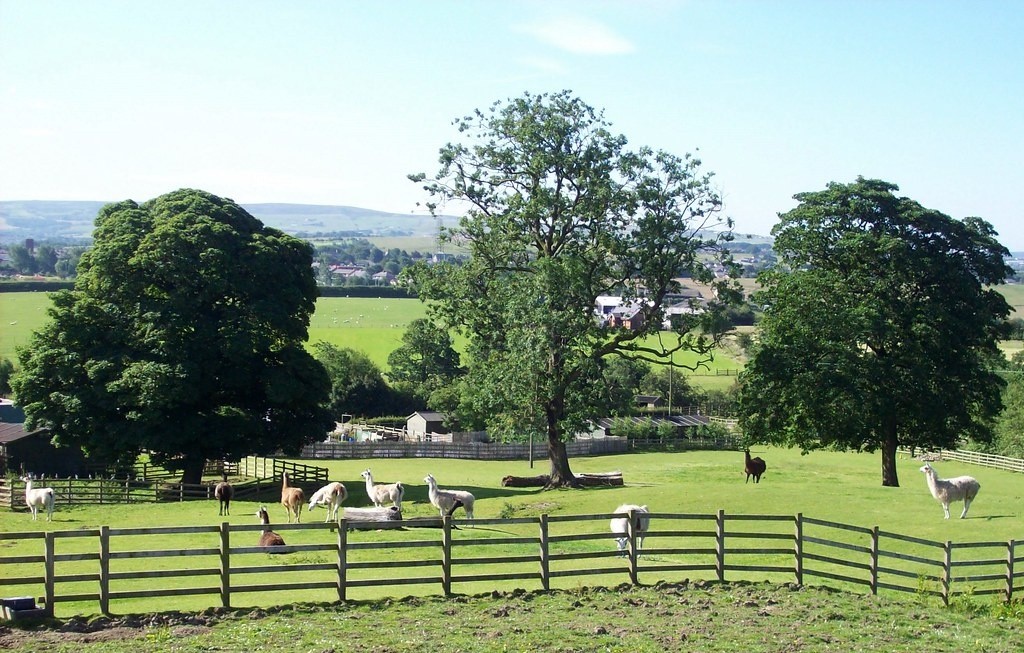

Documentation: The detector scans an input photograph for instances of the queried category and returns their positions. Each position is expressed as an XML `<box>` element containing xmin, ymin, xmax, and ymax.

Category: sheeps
<box><xmin>610</xmin><ymin>503</ymin><xmax>649</xmax><ymax>559</ymax></box>
